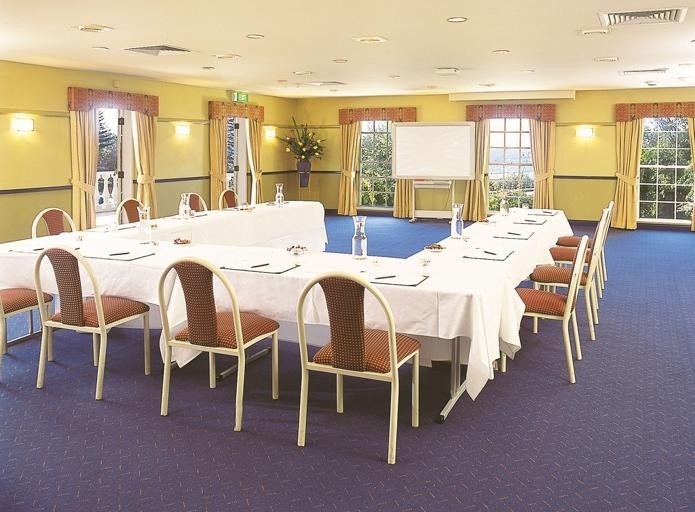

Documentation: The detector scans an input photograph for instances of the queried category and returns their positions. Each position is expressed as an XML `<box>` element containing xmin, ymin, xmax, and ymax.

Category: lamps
<box><xmin>7</xmin><ymin>118</ymin><xmax>37</xmax><ymax>134</ymax></box>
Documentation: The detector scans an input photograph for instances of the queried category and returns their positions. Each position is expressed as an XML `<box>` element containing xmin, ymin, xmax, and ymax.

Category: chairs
<box><xmin>27</xmin><ymin>187</ymin><xmax>241</xmax><ymax>240</ymax></box>
<box><xmin>151</xmin><ymin>258</ymin><xmax>281</xmax><ymax>437</ymax></box>
<box><xmin>500</xmin><ymin>201</ymin><xmax>618</xmax><ymax>385</ymax></box>
<box><xmin>27</xmin><ymin>246</ymin><xmax>151</xmax><ymax>403</ymax></box>
<box><xmin>294</xmin><ymin>272</ymin><xmax>424</xmax><ymax>474</ymax></box>
<box><xmin>0</xmin><ymin>284</ymin><xmax>55</xmax><ymax>364</ymax></box>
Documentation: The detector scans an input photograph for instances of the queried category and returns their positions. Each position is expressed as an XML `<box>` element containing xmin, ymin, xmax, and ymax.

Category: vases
<box><xmin>294</xmin><ymin>157</ymin><xmax>313</xmax><ymax>187</ymax></box>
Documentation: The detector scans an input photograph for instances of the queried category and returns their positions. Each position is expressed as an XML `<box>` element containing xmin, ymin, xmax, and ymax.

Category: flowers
<box><xmin>271</xmin><ymin>114</ymin><xmax>328</xmax><ymax>161</ymax></box>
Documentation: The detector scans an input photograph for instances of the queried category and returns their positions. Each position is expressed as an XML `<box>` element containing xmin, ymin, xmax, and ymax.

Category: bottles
<box><xmin>178</xmin><ymin>193</ymin><xmax>193</xmax><ymax>222</ymax></box>
<box><xmin>137</xmin><ymin>205</ymin><xmax>152</xmax><ymax>243</ymax></box>
<box><xmin>275</xmin><ymin>183</ymin><xmax>285</xmax><ymax>209</ymax></box>
<box><xmin>449</xmin><ymin>203</ymin><xmax>466</xmax><ymax>239</ymax></box>
<box><xmin>499</xmin><ymin>197</ymin><xmax>510</xmax><ymax>216</ymax></box>
<box><xmin>351</xmin><ymin>216</ymin><xmax>369</xmax><ymax>274</ymax></box>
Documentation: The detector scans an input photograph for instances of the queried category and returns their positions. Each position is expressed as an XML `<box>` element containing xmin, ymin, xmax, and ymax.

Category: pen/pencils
<box><xmin>110</xmin><ymin>252</ymin><xmax>129</xmax><ymax>256</ymax></box>
<box><xmin>508</xmin><ymin>232</ymin><xmax>520</xmax><ymax>235</ymax></box>
<box><xmin>34</xmin><ymin>248</ymin><xmax>44</xmax><ymax>251</ymax></box>
<box><xmin>543</xmin><ymin>211</ymin><xmax>552</xmax><ymax>214</ymax></box>
<box><xmin>376</xmin><ymin>276</ymin><xmax>396</xmax><ymax>279</ymax></box>
<box><xmin>525</xmin><ymin>220</ymin><xmax>536</xmax><ymax>222</ymax></box>
<box><xmin>251</xmin><ymin>264</ymin><xmax>269</xmax><ymax>268</ymax></box>
<box><xmin>483</xmin><ymin>251</ymin><xmax>496</xmax><ymax>255</ymax></box>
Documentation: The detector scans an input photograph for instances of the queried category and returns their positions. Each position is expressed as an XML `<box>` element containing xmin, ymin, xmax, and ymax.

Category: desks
<box><xmin>0</xmin><ymin>234</ymin><xmax>504</xmax><ymax>425</ymax></box>
<box><xmin>408</xmin><ymin>203</ymin><xmax>575</xmax><ymax>376</ymax></box>
<box><xmin>57</xmin><ymin>198</ymin><xmax>329</xmax><ymax>254</ymax></box>
